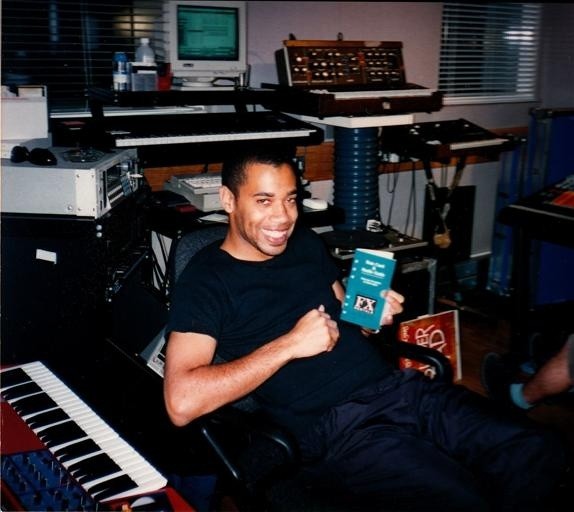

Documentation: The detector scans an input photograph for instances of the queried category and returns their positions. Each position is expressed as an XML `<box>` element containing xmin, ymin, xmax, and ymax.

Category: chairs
<box><xmin>164</xmin><ymin>224</ymin><xmax>454</xmax><ymax>511</ymax></box>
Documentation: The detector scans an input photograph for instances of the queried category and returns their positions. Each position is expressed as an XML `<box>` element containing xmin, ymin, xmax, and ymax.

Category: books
<box><xmin>338</xmin><ymin>247</ymin><xmax>398</xmax><ymax>333</ymax></box>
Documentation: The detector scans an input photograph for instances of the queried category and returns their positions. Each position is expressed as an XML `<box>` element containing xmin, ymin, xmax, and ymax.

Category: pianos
<box><xmin>0</xmin><ymin>361</ymin><xmax>197</xmax><ymax>512</ymax></box>
<box><xmin>49</xmin><ymin>110</ymin><xmax>324</xmax><ymax>170</ymax></box>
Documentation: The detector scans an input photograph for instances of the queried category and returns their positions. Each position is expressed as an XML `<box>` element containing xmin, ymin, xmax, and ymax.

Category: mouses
<box><xmin>28</xmin><ymin>147</ymin><xmax>57</xmax><ymax>166</ymax></box>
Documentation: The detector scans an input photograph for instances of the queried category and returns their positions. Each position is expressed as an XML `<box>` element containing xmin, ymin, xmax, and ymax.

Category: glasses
<box><xmin>10</xmin><ymin>146</ymin><xmax>57</xmax><ymax>166</ymax></box>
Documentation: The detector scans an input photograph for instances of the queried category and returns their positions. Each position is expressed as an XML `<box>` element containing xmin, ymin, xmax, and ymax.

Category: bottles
<box><xmin>114</xmin><ymin>37</ymin><xmax>157</xmax><ymax>93</ymax></box>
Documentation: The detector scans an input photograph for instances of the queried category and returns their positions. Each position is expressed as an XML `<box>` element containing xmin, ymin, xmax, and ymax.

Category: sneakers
<box><xmin>480</xmin><ymin>351</ymin><xmax>531</xmax><ymax>419</ymax></box>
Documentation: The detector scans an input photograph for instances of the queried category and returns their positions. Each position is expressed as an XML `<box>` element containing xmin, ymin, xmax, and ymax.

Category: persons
<box><xmin>161</xmin><ymin>137</ymin><xmax>573</xmax><ymax>509</ymax></box>
<box><xmin>485</xmin><ymin>325</ymin><xmax>573</xmax><ymax>416</ymax></box>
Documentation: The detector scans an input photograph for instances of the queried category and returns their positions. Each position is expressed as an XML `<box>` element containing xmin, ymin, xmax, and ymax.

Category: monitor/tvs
<box><xmin>164</xmin><ymin>0</ymin><xmax>248</xmax><ymax>91</ymax></box>
